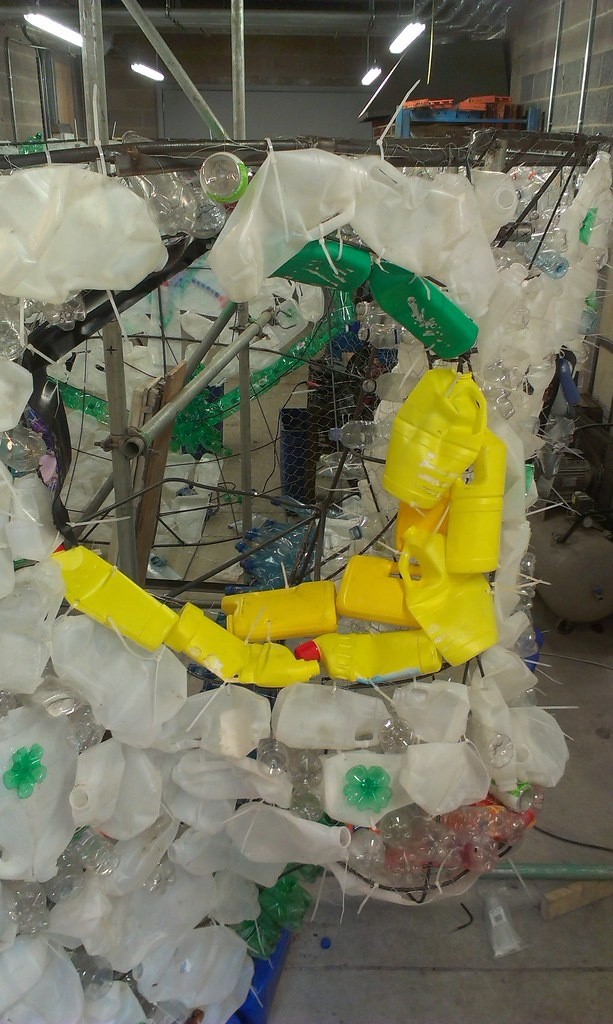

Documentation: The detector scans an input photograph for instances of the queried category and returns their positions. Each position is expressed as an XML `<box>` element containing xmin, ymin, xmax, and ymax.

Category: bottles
<box><xmin>0</xmin><ymin>131</ymin><xmax>613</xmax><ymax>1024</ymax></box>
